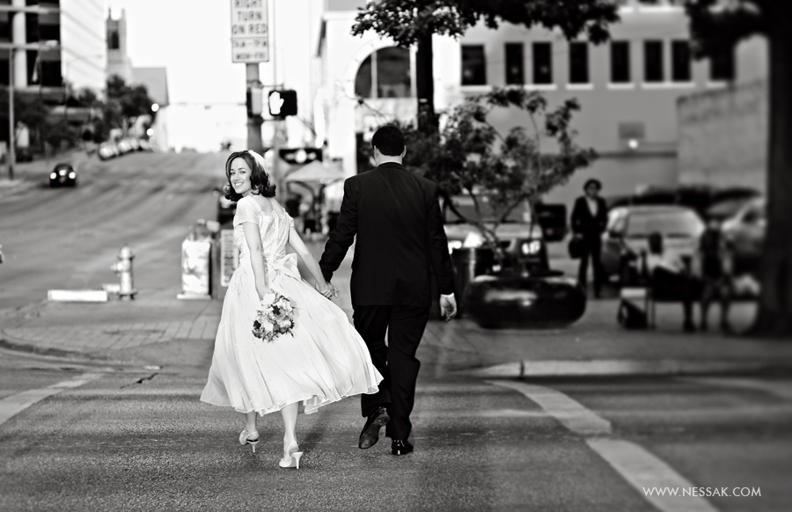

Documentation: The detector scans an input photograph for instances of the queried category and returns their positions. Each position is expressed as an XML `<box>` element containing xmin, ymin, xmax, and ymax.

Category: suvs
<box><xmin>441</xmin><ymin>195</ymin><xmax>550</xmax><ymax>277</ymax></box>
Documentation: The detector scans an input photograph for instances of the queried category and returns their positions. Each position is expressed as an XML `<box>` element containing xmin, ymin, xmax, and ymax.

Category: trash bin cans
<box><xmin>180</xmin><ymin>240</ymin><xmax>209</xmax><ymax>295</ymax></box>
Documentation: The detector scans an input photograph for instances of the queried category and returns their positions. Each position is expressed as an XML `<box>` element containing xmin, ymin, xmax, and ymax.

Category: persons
<box><xmin>571</xmin><ymin>179</ymin><xmax>608</xmax><ymax>299</ymax></box>
<box><xmin>199</xmin><ymin>150</ymin><xmax>384</xmax><ymax>470</ymax></box>
<box><xmin>637</xmin><ymin>234</ymin><xmax>702</xmax><ymax>333</ymax></box>
<box><xmin>693</xmin><ymin>218</ymin><xmax>737</xmax><ymax>332</ymax></box>
<box><xmin>316</xmin><ymin>126</ymin><xmax>459</xmax><ymax>455</ymax></box>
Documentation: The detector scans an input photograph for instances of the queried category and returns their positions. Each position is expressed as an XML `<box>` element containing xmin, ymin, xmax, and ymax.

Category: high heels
<box><xmin>278</xmin><ymin>441</ymin><xmax>303</xmax><ymax>469</ymax></box>
<box><xmin>239</xmin><ymin>429</ymin><xmax>260</xmax><ymax>453</ymax></box>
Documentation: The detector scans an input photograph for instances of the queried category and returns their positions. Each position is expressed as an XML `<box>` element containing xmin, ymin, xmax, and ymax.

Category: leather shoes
<box><xmin>359</xmin><ymin>406</ymin><xmax>390</xmax><ymax>449</ymax></box>
<box><xmin>391</xmin><ymin>439</ymin><xmax>413</xmax><ymax>455</ymax></box>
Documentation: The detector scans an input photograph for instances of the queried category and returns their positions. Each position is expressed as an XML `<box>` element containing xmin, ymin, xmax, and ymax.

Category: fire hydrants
<box><xmin>112</xmin><ymin>246</ymin><xmax>138</xmax><ymax>300</ymax></box>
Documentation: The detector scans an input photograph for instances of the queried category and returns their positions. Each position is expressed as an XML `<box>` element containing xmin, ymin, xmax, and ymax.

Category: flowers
<box><xmin>252</xmin><ymin>292</ymin><xmax>297</xmax><ymax>342</ymax></box>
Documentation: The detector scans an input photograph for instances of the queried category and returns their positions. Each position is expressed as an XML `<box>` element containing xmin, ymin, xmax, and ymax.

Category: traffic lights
<box><xmin>268</xmin><ymin>90</ymin><xmax>298</xmax><ymax>118</ymax></box>
<box><xmin>278</xmin><ymin>147</ymin><xmax>323</xmax><ymax>164</ymax></box>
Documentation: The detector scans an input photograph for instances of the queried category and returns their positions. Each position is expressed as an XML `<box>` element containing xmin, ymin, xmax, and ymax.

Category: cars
<box><xmin>723</xmin><ymin>200</ymin><xmax>767</xmax><ymax>271</ymax></box>
<box><xmin>601</xmin><ymin>204</ymin><xmax>706</xmax><ymax>284</ymax></box>
<box><xmin>212</xmin><ymin>151</ymin><xmax>344</xmax><ymax>225</ymax></box>
<box><xmin>50</xmin><ymin>163</ymin><xmax>77</xmax><ymax>187</ymax></box>
